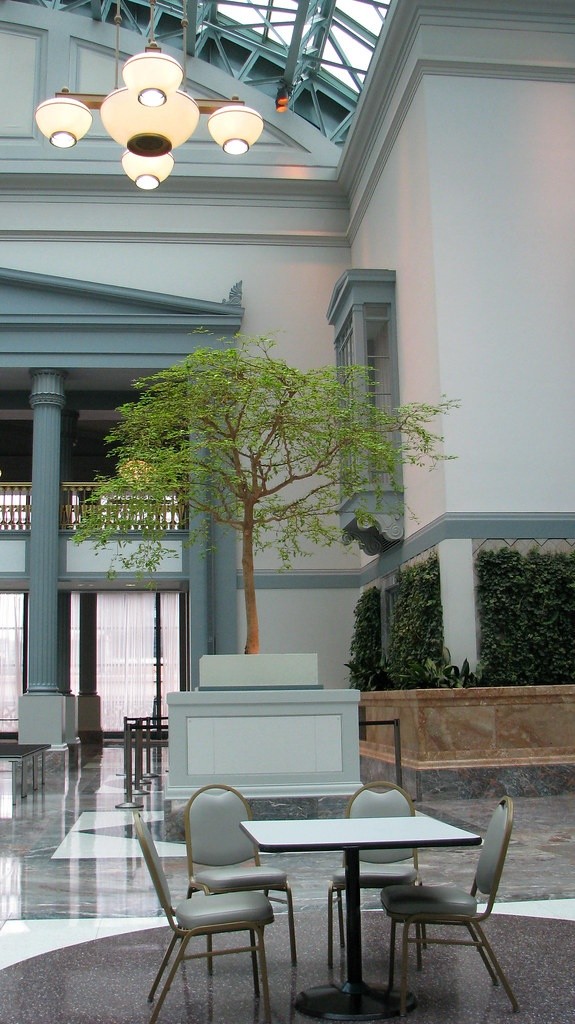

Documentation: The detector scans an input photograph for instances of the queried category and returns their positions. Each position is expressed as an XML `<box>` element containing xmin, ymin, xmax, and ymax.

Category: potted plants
<box><xmin>63</xmin><ymin>324</ymin><xmax>463</xmax><ymax>689</ymax></box>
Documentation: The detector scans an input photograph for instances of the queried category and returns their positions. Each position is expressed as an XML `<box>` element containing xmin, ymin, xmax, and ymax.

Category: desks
<box><xmin>240</xmin><ymin>818</ymin><xmax>483</xmax><ymax>1021</ymax></box>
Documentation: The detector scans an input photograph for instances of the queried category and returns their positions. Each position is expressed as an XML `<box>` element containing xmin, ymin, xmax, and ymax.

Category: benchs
<box><xmin>0</xmin><ymin>744</ymin><xmax>51</xmax><ymax>805</ymax></box>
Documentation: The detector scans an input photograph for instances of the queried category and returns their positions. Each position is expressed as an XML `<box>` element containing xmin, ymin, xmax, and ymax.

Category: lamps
<box><xmin>33</xmin><ymin>0</ymin><xmax>263</xmax><ymax>191</ymax></box>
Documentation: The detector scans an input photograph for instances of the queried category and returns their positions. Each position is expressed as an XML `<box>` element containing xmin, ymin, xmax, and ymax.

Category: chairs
<box><xmin>133</xmin><ymin>810</ymin><xmax>274</xmax><ymax>1024</ymax></box>
<box><xmin>380</xmin><ymin>795</ymin><xmax>519</xmax><ymax>1016</ymax></box>
<box><xmin>328</xmin><ymin>782</ymin><xmax>427</xmax><ymax>972</ymax></box>
<box><xmin>187</xmin><ymin>784</ymin><xmax>297</xmax><ymax>975</ymax></box>
